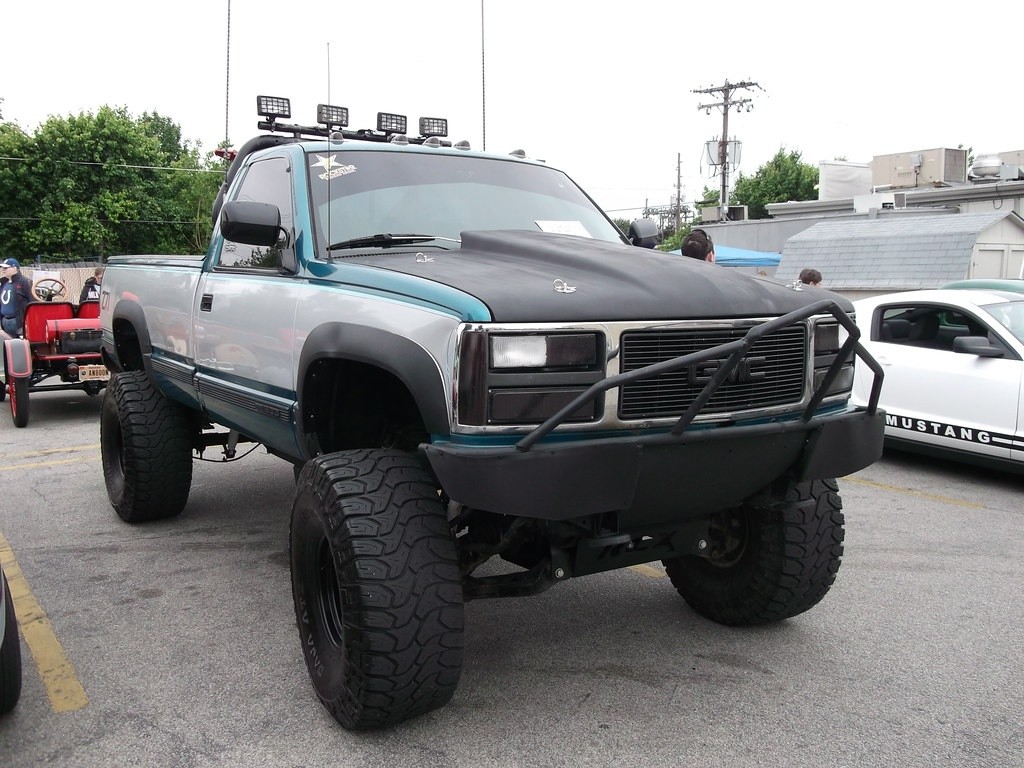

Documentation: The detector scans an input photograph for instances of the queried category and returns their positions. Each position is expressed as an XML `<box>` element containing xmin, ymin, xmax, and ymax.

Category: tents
<box><xmin>670</xmin><ymin>244</ymin><xmax>780</xmax><ymax>267</ymax></box>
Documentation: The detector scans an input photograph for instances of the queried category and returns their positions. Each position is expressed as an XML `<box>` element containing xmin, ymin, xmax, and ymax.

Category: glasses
<box><xmin>96</xmin><ymin>275</ymin><xmax>102</xmax><ymax>279</ymax></box>
<box><xmin>691</xmin><ymin>228</ymin><xmax>709</xmax><ymax>251</ymax></box>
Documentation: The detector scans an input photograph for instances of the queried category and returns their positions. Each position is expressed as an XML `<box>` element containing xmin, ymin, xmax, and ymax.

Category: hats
<box><xmin>0</xmin><ymin>258</ymin><xmax>19</xmax><ymax>267</ymax></box>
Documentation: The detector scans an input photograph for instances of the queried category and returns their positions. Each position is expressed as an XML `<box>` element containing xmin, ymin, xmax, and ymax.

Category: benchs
<box><xmin>884</xmin><ymin>319</ymin><xmax>968</xmax><ymax>349</ymax></box>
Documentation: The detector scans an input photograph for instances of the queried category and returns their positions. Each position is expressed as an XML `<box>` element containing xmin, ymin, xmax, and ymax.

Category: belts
<box><xmin>3</xmin><ymin>315</ymin><xmax>16</xmax><ymax>319</ymax></box>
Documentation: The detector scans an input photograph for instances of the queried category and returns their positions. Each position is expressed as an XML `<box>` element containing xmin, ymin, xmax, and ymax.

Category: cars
<box><xmin>829</xmin><ymin>288</ymin><xmax>1023</xmax><ymax>463</ymax></box>
<box><xmin>2</xmin><ymin>274</ymin><xmax>114</xmax><ymax>427</ymax></box>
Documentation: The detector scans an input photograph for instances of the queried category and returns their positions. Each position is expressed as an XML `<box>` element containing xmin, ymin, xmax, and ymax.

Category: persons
<box><xmin>79</xmin><ymin>268</ymin><xmax>104</xmax><ymax>303</ymax></box>
<box><xmin>0</xmin><ymin>258</ymin><xmax>36</xmax><ymax>339</ymax></box>
<box><xmin>798</xmin><ymin>268</ymin><xmax>822</xmax><ymax>287</ymax></box>
<box><xmin>680</xmin><ymin>228</ymin><xmax>715</xmax><ymax>263</ymax></box>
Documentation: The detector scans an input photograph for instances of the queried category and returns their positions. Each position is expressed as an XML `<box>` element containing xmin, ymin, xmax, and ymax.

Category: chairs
<box><xmin>22</xmin><ymin>300</ymin><xmax>100</xmax><ymax>342</ymax></box>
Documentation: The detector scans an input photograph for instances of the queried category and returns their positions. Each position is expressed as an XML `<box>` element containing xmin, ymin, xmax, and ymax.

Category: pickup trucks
<box><xmin>94</xmin><ymin>93</ymin><xmax>888</xmax><ymax>733</ymax></box>
